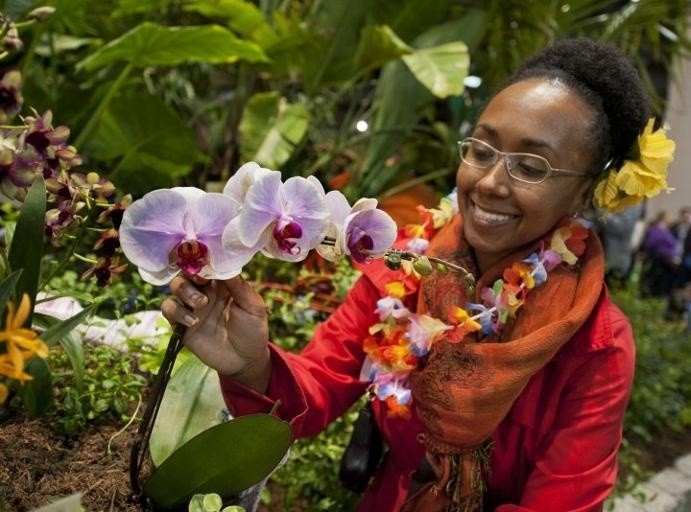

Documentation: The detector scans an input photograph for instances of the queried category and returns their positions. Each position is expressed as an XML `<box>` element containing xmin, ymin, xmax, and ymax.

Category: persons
<box><xmin>597</xmin><ymin>198</ymin><xmax>691</xmax><ymax>321</ymax></box>
<box><xmin>159</xmin><ymin>37</ymin><xmax>649</xmax><ymax>512</ymax></box>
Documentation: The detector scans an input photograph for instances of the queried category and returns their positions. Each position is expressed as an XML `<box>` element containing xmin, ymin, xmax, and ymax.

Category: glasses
<box><xmin>457</xmin><ymin>137</ymin><xmax>593</xmax><ymax>184</ymax></box>
